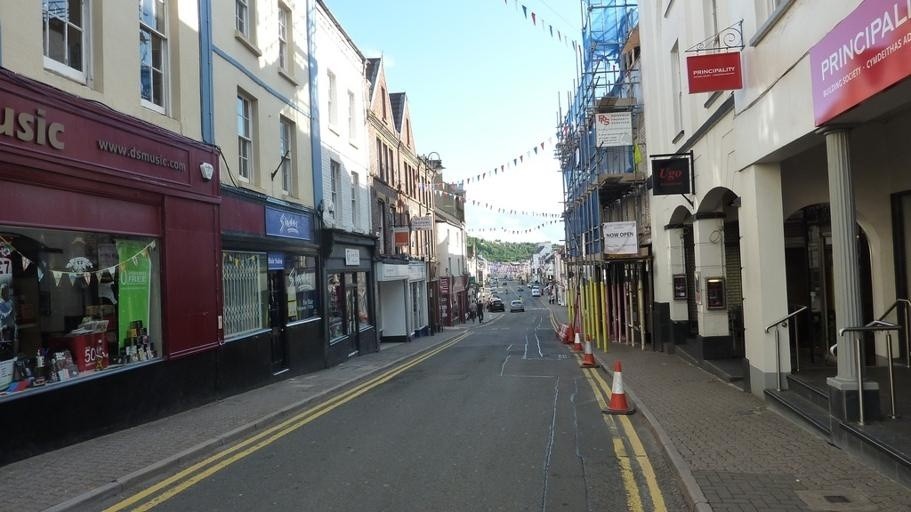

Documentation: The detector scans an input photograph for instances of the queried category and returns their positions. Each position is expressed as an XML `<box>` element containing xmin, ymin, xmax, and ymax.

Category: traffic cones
<box><xmin>572</xmin><ymin>327</ymin><xmax>582</xmax><ymax>351</ymax></box>
<box><xmin>580</xmin><ymin>333</ymin><xmax>598</xmax><ymax>369</ymax></box>
<box><xmin>567</xmin><ymin>324</ymin><xmax>574</xmax><ymax>343</ymax></box>
<box><xmin>601</xmin><ymin>360</ymin><xmax>637</xmax><ymax>415</ymax></box>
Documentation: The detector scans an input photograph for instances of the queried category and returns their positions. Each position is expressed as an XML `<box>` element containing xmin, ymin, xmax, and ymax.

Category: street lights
<box><xmin>425</xmin><ymin>151</ymin><xmax>445</xmax><ymax>215</ymax></box>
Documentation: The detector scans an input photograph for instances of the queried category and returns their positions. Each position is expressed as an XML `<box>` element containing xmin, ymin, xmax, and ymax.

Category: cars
<box><xmin>488</xmin><ymin>280</ymin><xmax>531</xmax><ymax>298</ymax></box>
<box><xmin>510</xmin><ymin>300</ymin><xmax>523</xmax><ymax>312</ymax></box>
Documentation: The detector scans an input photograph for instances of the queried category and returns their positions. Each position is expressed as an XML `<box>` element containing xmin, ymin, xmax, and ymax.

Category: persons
<box><xmin>469</xmin><ymin>299</ymin><xmax>477</xmax><ymax>322</ymax></box>
<box><xmin>452</xmin><ymin>300</ymin><xmax>459</xmax><ymax>317</ymax></box>
<box><xmin>475</xmin><ymin>300</ymin><xmax>484</xmax><ymax>323</ymax></box>
<box><xmin>547</xmin><ymin>292</ymin><xmax>555</xmax><ymax>304</ymax></box>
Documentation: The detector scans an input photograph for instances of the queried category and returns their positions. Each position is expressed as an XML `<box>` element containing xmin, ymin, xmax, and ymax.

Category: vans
<box><xmin>531</xmin><ymin>286</ymin><xmax>542</xmax><ymax>297</ymax></box>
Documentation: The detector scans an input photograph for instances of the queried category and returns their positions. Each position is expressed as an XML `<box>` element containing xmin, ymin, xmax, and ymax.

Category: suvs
<box><xmin>487</xmin><ymin>298</ymin><xmax>504</xmax><ymax>312</ymax></box>
